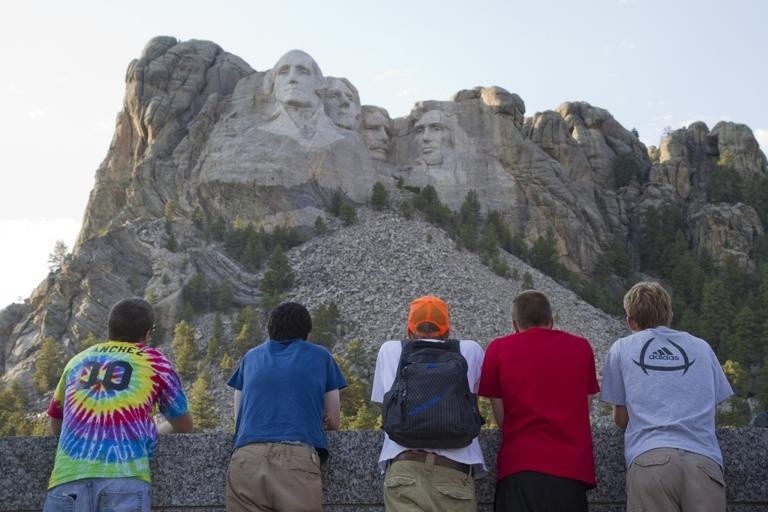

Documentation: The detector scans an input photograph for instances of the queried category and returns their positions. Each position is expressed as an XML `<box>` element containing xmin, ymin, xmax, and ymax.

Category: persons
<box><xmin>371</xmin><ymin>294</ymin><xmax>487</xmax><ymax>512</ymax></box>
<box><xmin>221</xmin><ymin>302</ymin><xmax>348</xmax><ymax>511</ymax></box>
<box><xmin>318</xmin><ymin>73</ymin><xmax>358</xmax><ymax>130</ymax></box>
<box><xmin>258</xmin><ymin>49</ymin><xmax>339</xmax><ymax>149</ymax></box>
<box><xmin>358</xmin><ymin>106</ymin><xmax>392</xmax><ymax>159</ymax></box>
<box><xmin>475</xmin><ymin>290</ymin><xmax>601</xmax><ymax>512</ymax></box>
<box><xmin>409</xmin><ymin>99</ymin><xmax>449</xmax><ymax>165</ymax></box>
<box><xmin>36</xmin><ymin>296</ymin><xmax>195</xmax><ymax>512</ymax></box>
<box><xmin>592</xmin><ymin>280</ymin><xmax>739</xmax><ymax>511</ymax></box>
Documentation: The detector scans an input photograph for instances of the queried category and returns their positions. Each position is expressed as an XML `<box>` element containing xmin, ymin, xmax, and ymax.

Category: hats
<box><xmin>408</xmin><ymin>296</ymin><xmax>449</xmax><ymax>337</ymax></box>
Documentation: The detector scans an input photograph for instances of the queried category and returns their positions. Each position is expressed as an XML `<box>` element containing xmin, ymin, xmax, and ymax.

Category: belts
<box><xmin>390</xmin><ymin>450</ymin><xmax>475</xmax><ymax>477</ymax></box>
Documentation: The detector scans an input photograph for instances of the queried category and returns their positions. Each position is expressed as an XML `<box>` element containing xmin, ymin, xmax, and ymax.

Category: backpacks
<box><xmin>381</xmin><ymin>340</ymin><xmax>485</xmax><ymax>448</ymax></box>
